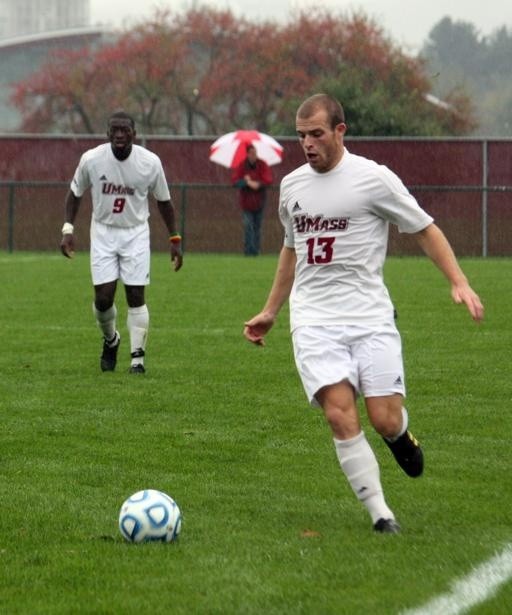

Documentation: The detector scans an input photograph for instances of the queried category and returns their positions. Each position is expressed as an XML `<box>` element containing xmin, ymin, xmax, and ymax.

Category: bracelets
<box><xmin>168</xmin><ymin>232</ymin><xmax>182</xmax><ymax>243</ymax></box>
<box><xmin>62</xmin><ymin>221</ymin><xmax>74</xmax><ymax>236</ymax></box>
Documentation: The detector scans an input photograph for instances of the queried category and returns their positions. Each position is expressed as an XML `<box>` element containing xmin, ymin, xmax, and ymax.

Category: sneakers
<box><xmin>130</xmin><ymin>347</ymin><xmax>145</xmax><ymax>373</ymax></box>
<box><xmin>382</xmin><ymin>429</ymin><xmax>424</xmax><ymax>477</ymax></box>
<box><xmin>99</xmin><ymin>330</ymin><xmax>120</xmax><ymax>372</ymax></box>
<box><xmin>373</xmin><ymin>518</ymin><xmax>400</xmax><ymax>534</ymax></box>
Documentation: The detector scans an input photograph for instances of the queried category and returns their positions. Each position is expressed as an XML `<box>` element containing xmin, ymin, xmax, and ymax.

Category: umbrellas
<box><xmin>210</xmin><ymin>130</ymin><xmax>285</xmax><ymax>168</ymax></box>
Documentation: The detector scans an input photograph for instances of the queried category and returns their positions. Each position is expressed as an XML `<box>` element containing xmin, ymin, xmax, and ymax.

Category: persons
<box><xmin>62</xmin><ymin>111</ymin><xmax>184</xmax><ymax>374</ymax></box>
<box><xmin>232</xmin><ymin>144</ymin><xmax>274</xmax><ymax>255</ymax></box>
<box><xmin>242</xmin><ymin>91</ymin><xmax>485</xmax><ymax>537</ymax></box>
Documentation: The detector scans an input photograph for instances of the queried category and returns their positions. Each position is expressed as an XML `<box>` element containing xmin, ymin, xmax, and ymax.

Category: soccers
<box><xmin>118</xmin><ymin>489</ymin><xmax>181</xmax><ymax>542</ymax></box>
